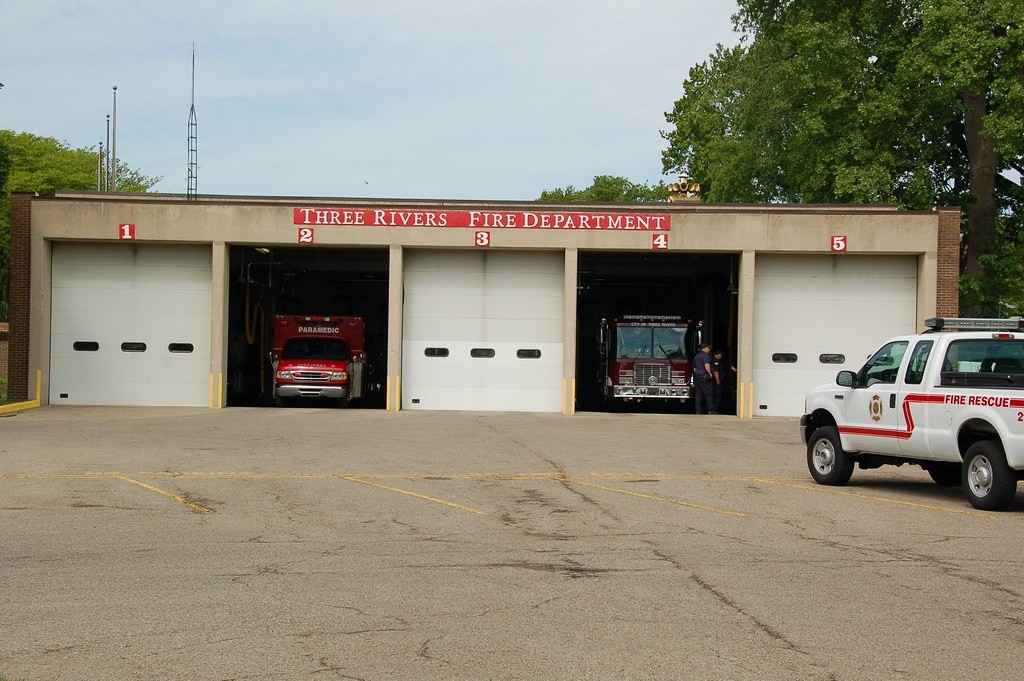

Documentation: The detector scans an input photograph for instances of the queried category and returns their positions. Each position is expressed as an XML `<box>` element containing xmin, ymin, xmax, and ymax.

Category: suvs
<box><xmin>799</xmin><ymin>316</ymin><xmax>1024</xmax><ymax>511</ymax></box>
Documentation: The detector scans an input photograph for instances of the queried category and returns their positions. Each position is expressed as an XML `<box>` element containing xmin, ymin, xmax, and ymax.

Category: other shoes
<box><xmin>708</xmin><ymin>409</ymin><xmax>718</xmax><ymax>414</ymax></box>
<box><xmin>696</xmin><ymin>410</ymin><xmax>703</xmax><ymax>414</ymax></box>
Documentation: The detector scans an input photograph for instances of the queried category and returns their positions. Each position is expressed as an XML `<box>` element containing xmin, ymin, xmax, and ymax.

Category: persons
<box><xmin>693</xmin><ymin>343</ymin><xmax>724</xmax><ymax>414</ymax></box>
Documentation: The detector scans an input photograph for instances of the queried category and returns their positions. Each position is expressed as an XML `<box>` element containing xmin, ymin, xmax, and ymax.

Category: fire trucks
<box><xmin>596</xmin><ymin>313</ymin><xmax>704</xmax><ymax>414</ymax></box>
<box><xmin>266</xmin><ymin>311</ymin><xmax>368</xmax><ymax>409</ymax></box>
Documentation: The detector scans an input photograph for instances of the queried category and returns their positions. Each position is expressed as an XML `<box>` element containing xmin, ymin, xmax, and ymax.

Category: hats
<box><xmin>701</xmin><ymin>343</ymin><xmax>712</xmax><ymax>349</ymax></box>
<box><xmin>714</xmin><ymin>350</ymin><xmax>725</xmax><ymax>355</ymax></box>
